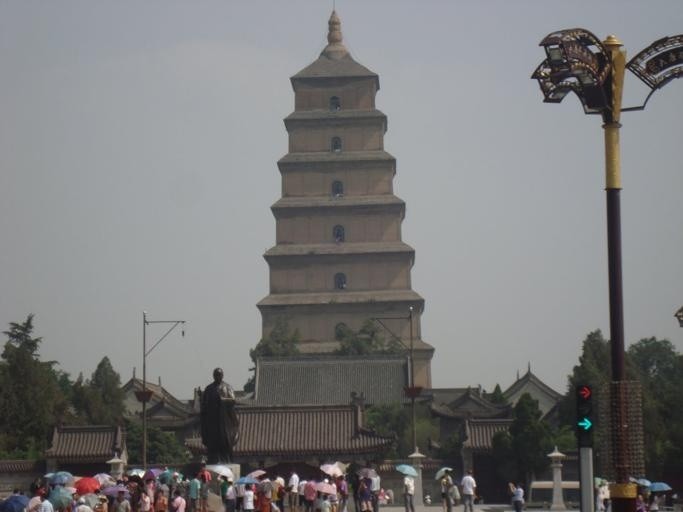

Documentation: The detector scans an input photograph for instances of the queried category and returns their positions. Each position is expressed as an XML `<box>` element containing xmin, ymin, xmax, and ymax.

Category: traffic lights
<box><xmin>575</xmin><ymin>384</ymin><xmax>595</xmax><ymax>446</ymax></box>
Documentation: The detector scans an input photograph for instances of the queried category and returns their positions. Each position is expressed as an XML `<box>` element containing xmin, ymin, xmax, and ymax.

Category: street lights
<box><xmin>528</xmin><ymin>27</ymin><xmax>682</xmax><ymax>512</ymax></box>
<box><xmin>135</xmin><ymin>309</ymin><xmax>190</xmax><ymax>468</ymax></box>
<box><xmin>370</xmin><ymin>306</ymin><xmax>424</xmax><ymax>461</ymax></box>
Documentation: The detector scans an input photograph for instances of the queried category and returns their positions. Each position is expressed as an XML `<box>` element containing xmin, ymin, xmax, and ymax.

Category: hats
<box><xmin>78</xmin><ymin>498</ymin><xmax>85</xmax><ymax>504</ymax></box>
<box><xmin>97</xmin><ymin>494</ymin><xmax>109</xmax><ymax>503</ymax></box>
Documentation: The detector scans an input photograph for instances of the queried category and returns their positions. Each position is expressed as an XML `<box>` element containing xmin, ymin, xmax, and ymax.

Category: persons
<box><xmin>508</xmin><ymin>482</ymin><xmax>526</xmax><ymax>512</ymax></box>
<box><xmin>352</xmin><ymin>475</ymin><xmax>431</xmax><ymax>512</ymax></box>
<box><xmin>198</xmin><ymin>368</ymin><xmax>240</xmax><ymax>465</ymax></box>
<box><xmin>441</xmin><ymin>468</ymin><xmax>476</xmax><ymax>512</ymax></box>
<box><xmin>1</xmin><ymin>465</ymin><xmax>348</xmax><ymax>512</ymax></box>
<box><xmin>597</xmin><ymin>478</ymin><xmax>683</xmax><ymax>512</ymax></box>
